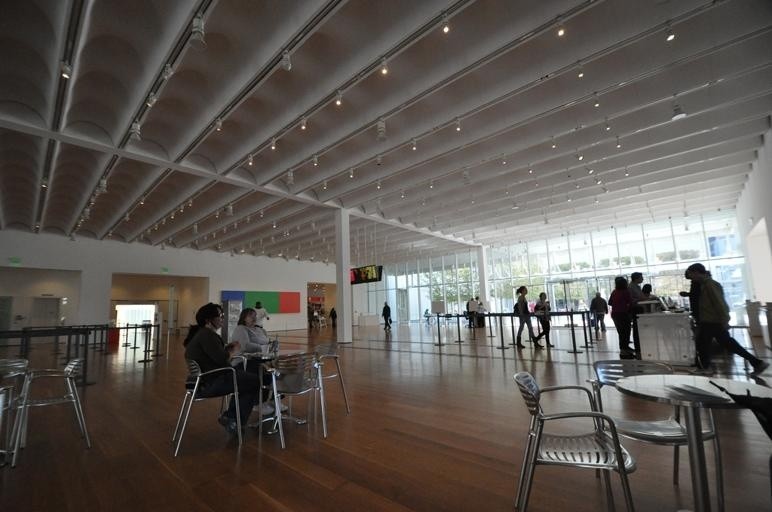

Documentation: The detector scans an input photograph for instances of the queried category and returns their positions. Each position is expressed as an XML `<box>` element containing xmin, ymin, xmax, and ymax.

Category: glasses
<box><xmin>208</xmin><ymin>312</ymin><xmax>226</xmax><ymax>319</ymax></box>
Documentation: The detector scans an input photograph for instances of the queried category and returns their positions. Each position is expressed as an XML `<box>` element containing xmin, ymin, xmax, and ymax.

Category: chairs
<box><xmin>512</xmin><ymin>369</ymin><xmax>640</xmax><ymax>509</ymax></box>
<box><xmin>584</xmin><ymin>358</ymin><xmax>726</xmax><ymax>508</ymax></box>
<box><xmin>172</xmin><ymin>342</ymin><xmax>352</xmax><ymax>455</ymax></box>
<box><xmin>1</xmin><ymin>347</ymin><xmax>92</xmax><ymax>472</ymax></box>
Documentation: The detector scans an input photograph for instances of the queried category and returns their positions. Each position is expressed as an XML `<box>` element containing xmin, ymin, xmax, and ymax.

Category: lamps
<box><xmin>34</xmin><ymin>11</ymin><xmax>693</xmax><ymax>266</ymax></box>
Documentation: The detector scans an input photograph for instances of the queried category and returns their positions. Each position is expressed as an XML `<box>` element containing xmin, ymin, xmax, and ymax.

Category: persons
<box><xmin>233</xmin><ymin>308</ymin><xmax>289</xmax><ymax>415</ymax></box>
<box><xmin>183</xmin><ymin>302</ymin><xmax>260</xmax><ymax>436</ymax></box>
<box><xmin>466</xmin><ymin>296</ymin><xmax>485</xmax><ymax>328</ymax></box>
<box><xmin>329</xmin><ymin>308</ymin><xmax>337</xmax><ymax>327</ymax></box>
<box><xmin>308</xmin><ymin>306</ymin><xmax>325</xmax><ymax>328</ymax></box>
<box><xmin>534</xmin><ymin>293</ymin><xmax>554</xmax><ymax>347</ymax></box>
<box><xmin>382</xmin><ymin>302</ymin><xmax>391</xmax><ymax>329</ymax></box>
<box><xmin>608</xmin><ymin>272</ymin><xmax>652</xmax><ymax>358</ymax></box>
<box><xmin>589</xmin><ymin>292</ymin><xmax>608</xmax><ymax>331</ymax></box>
<box><xmin>425</xmin><ymin>309</ymin><xmax>430</xmax><ymax>325</ymax></box>
<box><xmin>254</xmin><ymin>302</ymin><xmax>270</xmax><ymax>328</ymax></box>
<box><xmin>517</xmin><ymin>286</ymin><xmax>544</xmax><ymax>348</ymax></box>
<box><xmin>681</xmin><ymin>264</ymin><xmax>770</xmax><ymax>377</ymax></box>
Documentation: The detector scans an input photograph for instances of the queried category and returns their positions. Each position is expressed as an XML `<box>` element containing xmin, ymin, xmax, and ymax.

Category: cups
<box><xmin>272</xmin><ymin>340</ymin><xmax>279</xmax><ymax>352</ymax></box>
<box><xmin>260</xmin><ymin>344</ymin><xmax>270</xmax><ymax>356</ymax></box>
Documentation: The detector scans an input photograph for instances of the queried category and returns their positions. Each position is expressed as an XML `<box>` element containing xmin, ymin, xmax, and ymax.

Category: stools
<box><xmin>727</xmin><ymin>324</ymin><xmax>757</xmax><ymax>360</ymax></box>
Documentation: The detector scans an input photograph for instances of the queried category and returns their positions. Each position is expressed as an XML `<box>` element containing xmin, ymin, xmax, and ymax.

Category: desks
<box><xmin>611</xmin><ymin>372</ymin><xmax>769</xmax><ymax>512</ymax></box>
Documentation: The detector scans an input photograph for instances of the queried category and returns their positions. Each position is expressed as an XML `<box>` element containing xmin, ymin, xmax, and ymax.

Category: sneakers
<box><xmin>748</xmin><ymin>360</ymin><xmax>769</xmax><ymax>378</ymax></box>
<box><xmin>249</xmin><ymin>400</ymin><xmax>290</xmax><ymax>417</ymax></box>
<box><xmin>614</xmin><ymin>344</ymin><xmax>640</xmax><ymax>362</ymax></box>
<box><xmin>218</xmin><ymin>413</ymin><xmax>246</xmax><ymax>439</ymax></box>
<box><xmin>515</xmin><ymin>340</ymin><xmax>556</xmax><ymax>350</ymax></box>
<box><xmin>690</xmin><ymin>361</ymin><xmax>719</xmax><ymax>375</ymax></box>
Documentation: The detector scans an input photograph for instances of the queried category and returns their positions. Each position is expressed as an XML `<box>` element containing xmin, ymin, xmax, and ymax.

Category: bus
<box><xmin>555</xmin><ymin>297</ymin><xmax>585</xmax><ymax>312</ymax></box>
